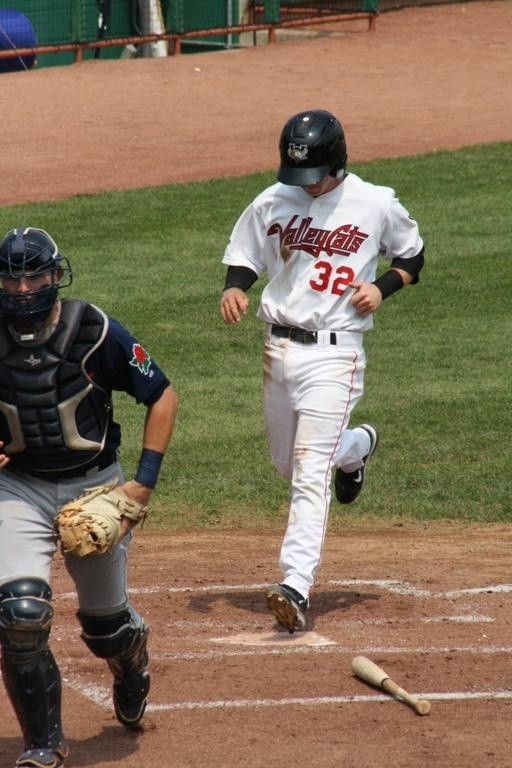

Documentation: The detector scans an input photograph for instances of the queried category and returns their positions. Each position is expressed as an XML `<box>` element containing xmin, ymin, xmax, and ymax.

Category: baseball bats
<box><xmin>351</xmin><ymin>656</ymin><xmax>432</xmax><ymax>715</ymax></box>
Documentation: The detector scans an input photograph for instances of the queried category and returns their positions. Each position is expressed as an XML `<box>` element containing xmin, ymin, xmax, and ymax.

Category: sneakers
<box><xmin>108</xmin><ymin>651</ymin><xmax>151</xmax><ymax>726</ymax></box>
<box><xmin>332</xmin><ymin>424</ymin><xmax>378</xmax><ymax>503</ymax></box>
<box><xmin>16</xmin><ymin>748</ymin><xmax>66</xmax><ymax>768</ymax></box>
<box><xmin>268</xmin><ymin>584</ymin><xmax>309</xmax><ymax>635</ymax></box>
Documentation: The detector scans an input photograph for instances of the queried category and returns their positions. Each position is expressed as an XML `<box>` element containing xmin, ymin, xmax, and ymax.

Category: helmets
<box><xmin>0</xmin><ymin>227</ymin><xmax>63</xmax><ymax>325</ymax></box>
<box><xmin>276</xmin><ymin>109</ymin><xmax>346</xmax><ymax>187</ymax></box>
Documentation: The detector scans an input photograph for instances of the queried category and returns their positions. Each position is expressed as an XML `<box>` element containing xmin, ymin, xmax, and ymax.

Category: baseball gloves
<box><xmin>54</xmin><ymin>478</ymin><xmax>152</xmax><ymax>558</ymax></box>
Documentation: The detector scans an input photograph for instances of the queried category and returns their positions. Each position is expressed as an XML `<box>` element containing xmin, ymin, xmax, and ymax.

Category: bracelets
<box><xmin>371</xmin><ymin>270</ymin><xmax>404</xmax><ymax>302</ymax></box>
<box><xmin>135</xmin><ymin>446</ymin><xmax>165</xmax><ymax>490</ymax></box>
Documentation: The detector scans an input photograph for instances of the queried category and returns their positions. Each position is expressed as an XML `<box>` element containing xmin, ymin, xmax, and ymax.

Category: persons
<box><xmin>221</xmin><ymin>109</ymin><xmax>425</xmax><ymax>631</ymax></box>
<box><xmin>0</xmin><ymin>226</ymin><xmax>177</xmax><ymax>768</ymax></box>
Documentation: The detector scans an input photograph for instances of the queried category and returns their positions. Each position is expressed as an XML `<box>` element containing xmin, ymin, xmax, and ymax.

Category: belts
<box><xmin>267</xmin><ymin>322</ymin><xmax>335</xmax><ymax>345</ymax></box>
